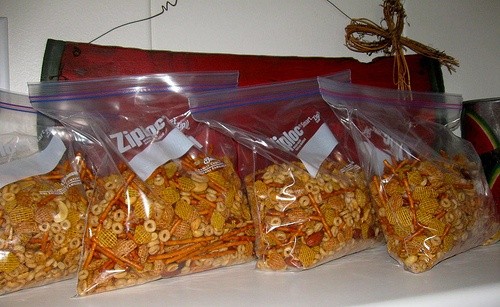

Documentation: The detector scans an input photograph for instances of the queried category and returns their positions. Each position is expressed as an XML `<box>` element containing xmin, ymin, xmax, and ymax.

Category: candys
<box><xmin>0</xmin><ymin>151</ymin><xmax>500</xmax><ymax>297</ymax></box>
